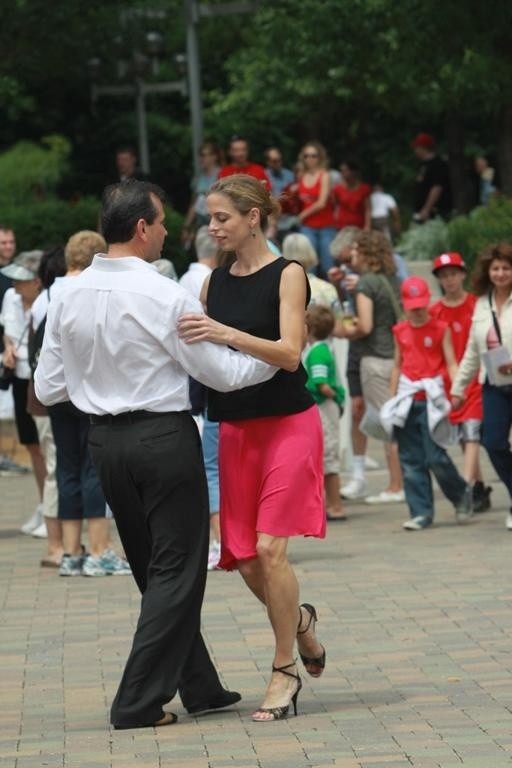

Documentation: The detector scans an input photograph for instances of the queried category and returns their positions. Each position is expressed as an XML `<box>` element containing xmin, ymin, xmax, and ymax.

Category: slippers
<box><xmin>325</xmin><ymin>508</ymin><xmax>349</xmax><ymax>521</ymax></box>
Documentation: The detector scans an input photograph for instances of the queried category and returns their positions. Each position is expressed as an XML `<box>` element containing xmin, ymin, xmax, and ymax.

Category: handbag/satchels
<box><xmin>0</xmin><ymin>362</ymin><xmax>12</xmax><ymax>390</ymax></box>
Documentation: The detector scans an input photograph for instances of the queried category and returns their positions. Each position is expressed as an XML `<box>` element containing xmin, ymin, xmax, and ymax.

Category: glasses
<box><xmin>303</xmin><ymin>152</ymin><xmax>318</xmax><ymax>157</ymax></box>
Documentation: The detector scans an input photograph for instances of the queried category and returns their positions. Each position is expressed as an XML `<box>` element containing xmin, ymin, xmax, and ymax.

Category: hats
<box><xmin>1</xmin><ymin>250</ymin><xmax>44</xmax><ymax>281</ymax></box>
<box><xmin>402</xmin><ymin>277</ymin><xmax>430</xmax><ymax>310</ymax></box>
<box><xmin>283</xmin><ymin>233</ymin><xmax>317</xmax><ymax>268</ymax></box>
<box><xmin>432</xmin><ymin>254</ymin><xmax>464</xmax><ymax>274</ymax></box>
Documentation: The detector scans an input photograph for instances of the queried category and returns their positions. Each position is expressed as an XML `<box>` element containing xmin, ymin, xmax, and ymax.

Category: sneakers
<box><xmin>403</xmin><ymin>516</ymin><xmax>430</xmax><ymax>530</ymax></box>
<box><xmin>206</xmin><ymin>540</ymin><xmax>222</xmax><ymax>570</ymax></box>
<box><xmin>339</xmin><ymin>479</ymin><xmax>366</xmax><ymax>499</ymax></box>
<box><xmin>456</xmin><ymin>486</ymin><xmax>473</xmax><ymax>522</ymax></box>
<box><xmin>1</xmin><ymin>459</ymin><xmax>34</xmax><ymax>477</ymax></box>
<box><xmin>188</xmin><ymin>686</ymin><xmax>241</xmax><ymax>713</ymax></box>
<box><xmin>20</xmin><ymin>505</ymin><xmax>43</xmax><ymax>532</ymax></box>
<box><xmin>365</xmin><ymin>490</ymin><xmax>405</xmax><ymax>502</ymax></box>
<box><xmin>59</xmin><ymin>549</ymin><xmax>90</xmax><ymax>577</ymax></box>
<box><xmin>473</xmin><ymin>481</ymin><xmax>492</xmax><ymax>512</ymax></box>
<box><xmin>114</xmin><ymin>711</ymin><xmax>177</xmax><ymax>728</ymax></box>
<box><xmin>83</xmin><ymin>548</ymin><xmax>133</xmax><ymax>576</ymax></box>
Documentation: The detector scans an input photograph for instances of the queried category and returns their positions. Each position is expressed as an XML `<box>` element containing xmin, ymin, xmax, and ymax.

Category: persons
<box><xmin>2</xmin><ymin>130</ymin><xmax>511</xmax><ymax>580</ymax></box>
<box><xmin>32</xmin><ymin>177</ymin><xmax>311</xmax><ymax>732</ymax></box>
<box><xmin>177</xmin><ymin>172</ymin><xmax>325</xmax><ymax>722</ymax></box>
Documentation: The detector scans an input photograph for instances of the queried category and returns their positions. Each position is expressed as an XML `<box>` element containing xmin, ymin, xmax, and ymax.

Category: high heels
<box><xmin>298</xmin><ymin>603</ymin><xmax>327</xmax><ymax>678</ymax></box>
<box><xmin>252</xmin><ymin>662</ymin><xmax>301</xmax><ymax>722</ymax></box>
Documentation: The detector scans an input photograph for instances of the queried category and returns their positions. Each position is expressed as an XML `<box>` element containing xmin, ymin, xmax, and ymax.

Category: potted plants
<box><xmin>395</xmin><ymin>216</ymin><xmax>451</xmax><ymax>296</ymax></box>
